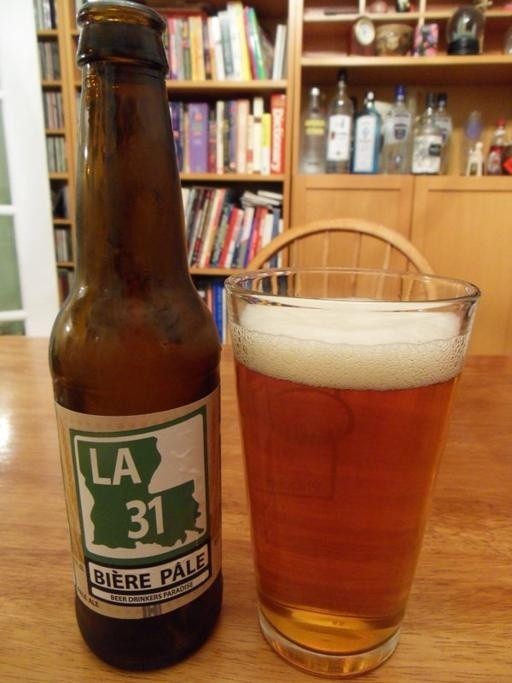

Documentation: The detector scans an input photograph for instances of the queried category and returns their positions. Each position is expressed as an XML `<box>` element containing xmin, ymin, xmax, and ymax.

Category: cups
<box><xmin>223</xmin><ymin>265</ymin><xmax>483</xmax><ymax>679</ymax></box>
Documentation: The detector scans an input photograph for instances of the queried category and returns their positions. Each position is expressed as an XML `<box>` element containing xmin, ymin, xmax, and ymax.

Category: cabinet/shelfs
<box><xmin>292</xmin><ymin>176</ymin><xmax>512</xmax><ymax>351</ymax></box>
<box><xmin>295</xmin><ymin>0</ymin><xmax>511</xmax><ymax>176</ymax></box>
<box><xmin>65</xmin><ymin>0</ymin><xmax>295</xmax><ymax>342</ymax></box>
<box><xmin>33</xmin><ymin>2</ymin><xmax>75</xmax><ymax>274</ymax></box>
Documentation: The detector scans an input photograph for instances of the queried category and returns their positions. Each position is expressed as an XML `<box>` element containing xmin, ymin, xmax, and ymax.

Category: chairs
<box><xmin>247</xmin><ymin>217</ymin><xmax>443</xmax><ymax>305</ymax></box>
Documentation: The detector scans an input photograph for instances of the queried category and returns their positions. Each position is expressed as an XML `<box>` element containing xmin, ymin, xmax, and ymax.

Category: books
<box><xmin>34</xmin><ymin>0</ymin><xmax>83</xmax><ymax>298</ymax></box>
<box><xmin>168</xmin><ymin>94</ymin><xmax>287</xmax><ymax>174</ymax></box>
<box><xmin>136</xmin><ymin>1</ymin><xmax>287</xmax><ymax>82</ymax></box>
<box><xmin>195</xmin><ymin>274</ymin><xmax>288</xmax><ymax>346</ymax></box>
<box><xmin>181</xmin><ymin>186</ymin><xmax>283</xmax><ymax>269</ymax></box>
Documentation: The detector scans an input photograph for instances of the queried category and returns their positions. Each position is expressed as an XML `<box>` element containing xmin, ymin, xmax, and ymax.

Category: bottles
<box><xmin>47</xmin><ymin>0</ymin><xmax>223</xmax><ymax>673</ymax></box>
<box><xmin>299</xmin><ymin>70</ymin><xmax>512</xmax><ymax>176</ymax></box>
